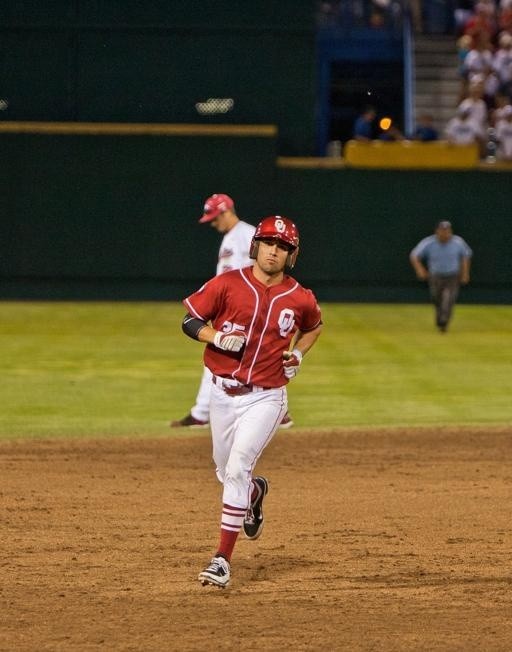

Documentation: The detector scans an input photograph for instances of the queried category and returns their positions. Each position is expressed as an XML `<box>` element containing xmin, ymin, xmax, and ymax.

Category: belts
<box><xmin>212</xmin><ymin>375</ymin><xmax>271</xmax><ymax>396</ymax></box>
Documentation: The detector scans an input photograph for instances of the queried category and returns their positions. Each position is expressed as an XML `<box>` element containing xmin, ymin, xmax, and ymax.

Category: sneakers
<box><xmin>279</xmin><ymin>410</ymin><xmax>294</xmax><ymax>427</ymax></box>
<box><xmin>197</xmin><ymin>554</ymin><xmax>231</xmax><ymax>589</ymax></box>
<box><xmin>243</xmin><ymin>475</ymin><xmax>269</xmax><ymax>540</ymax></box>
<box><xmin>170</xmin><ymin>413</ymin><xmax>210</xmax><ymax>430</ymax></box>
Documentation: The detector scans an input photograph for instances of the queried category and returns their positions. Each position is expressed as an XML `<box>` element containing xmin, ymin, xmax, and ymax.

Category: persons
<box><xmin>177</xmin><ymin>212</ymin><xmax>325</xmax><ymax>587</ymax></box>
<box><xmin>315</xmin><ymin>1</ymin><xmax>512</xmax><ymax>169</ymax></box>
<box><xmin>168</xmin><ymin>192</ymin><xmax>293</xmax><ymax>435</ymax></box>
<box><xmin>406</xmin><ymin>217</ymin><xmax>471</xmax><ymax>335</ymax></box>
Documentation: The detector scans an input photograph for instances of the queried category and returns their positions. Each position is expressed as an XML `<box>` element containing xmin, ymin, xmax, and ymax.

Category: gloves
<box><xmin>282</xmin><ymin>350</ymin><xmax>303</xmax><ymax>379</ymax></box>
<box><xmin>214</xmin><ymin>330</ymin><xmax>247</xmax><ymax>352</ymax></box>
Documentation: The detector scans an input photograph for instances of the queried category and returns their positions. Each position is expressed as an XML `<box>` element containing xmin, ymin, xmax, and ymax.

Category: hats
<box><xmin>199</xmin><ymin>193</ymin><xmax>235</xmax><ymax>224</ymax></box>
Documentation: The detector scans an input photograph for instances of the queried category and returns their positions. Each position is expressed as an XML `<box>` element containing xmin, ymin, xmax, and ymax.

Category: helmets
<box><xmin>249</xmin><ymin>215</ymin><xmax>301</xmax><ymax>269</ymax></box>
